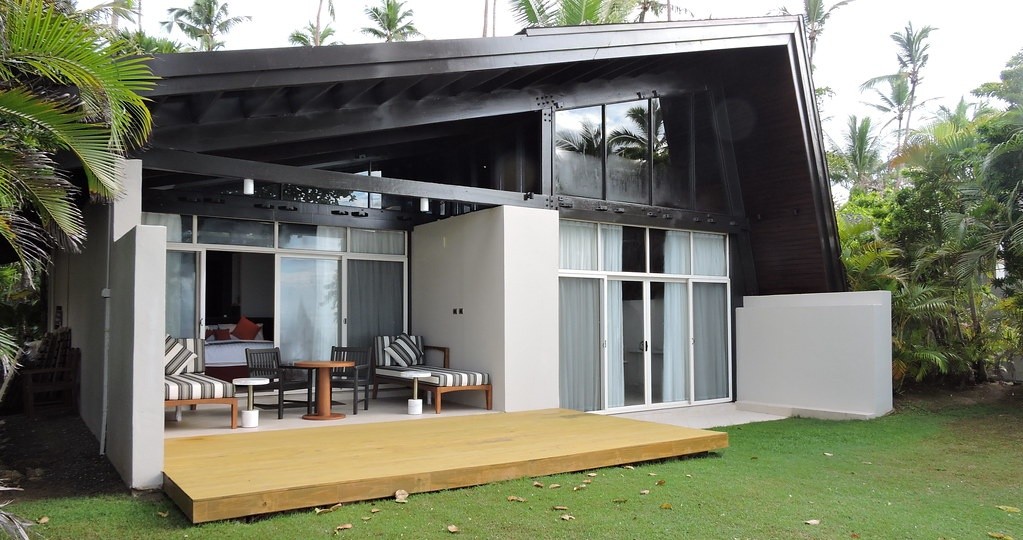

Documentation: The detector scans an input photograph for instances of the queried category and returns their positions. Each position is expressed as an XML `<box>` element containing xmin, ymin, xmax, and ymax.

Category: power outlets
<box><xmin>459</xmin><ymin>308</ymin><xmax>463</xmax><ymax>314</ymax></box>
<box><xmin>453</xmin><ymin>308</ymin><xmax>458</xmax><ymax>314</ymax></box>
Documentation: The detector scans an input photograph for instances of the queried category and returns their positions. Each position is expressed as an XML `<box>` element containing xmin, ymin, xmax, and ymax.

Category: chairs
<box><xmin>373</xmin><ymin>336</ymin><xmax>492</xmax><ymax>414</ymax></box>
<box><xmin>21</xmin><ymin>348</ymin><xmax>81</xmax><ymax>417</ymax></box>
<box><xmin>330</xmin><ymin>346</ymin><xmax>372</xmax><ymax>415</ymax></box>
<box><xmin>245</xmin><ymin>347</ymin><xmax>312</xmax><ymax>418</ymax></box>
<box><xmin>164</xmin><ymin>339</ymin><xmax>238</xmax><ymax>429</ymax></box>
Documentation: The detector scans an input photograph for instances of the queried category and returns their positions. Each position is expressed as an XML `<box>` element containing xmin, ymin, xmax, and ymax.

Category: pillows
<box><xmin>384</xmin><ymin>332</ymin><xmax>424</xmax><ymax>367</ymax></box>
<box><xmin>165</xmin><ymin>333</ymin><xmax>197</xmax><ymax>375</ymax></box>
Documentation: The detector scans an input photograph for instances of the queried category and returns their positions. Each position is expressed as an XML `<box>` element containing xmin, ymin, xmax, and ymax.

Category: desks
<box><xmin>294</xmin><ymin>361</ymin><xmax>355</xmax><ymax>420</ymax></box>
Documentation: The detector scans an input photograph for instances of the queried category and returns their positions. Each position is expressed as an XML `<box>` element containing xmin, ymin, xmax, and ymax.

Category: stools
<box><xmin>233</xmin><ymin>377</ymin><xmax>269</xmax><ymax>428</ymax></box>
<box><xmin>401</xmin><ymin>371</ymin><xmax>431</xmax><ymax>415</ymax></box>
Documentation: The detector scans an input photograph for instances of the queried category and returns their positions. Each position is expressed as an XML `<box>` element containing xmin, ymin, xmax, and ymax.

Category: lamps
<box><xmin>420</xmin><ymin>198</ymin><xmax>429</xmax><ymax>211</ymax></box>
<box><xmin>244</xmin><ymin>178</ymin><xmax>254</xmax><ymax>195</ymax></box>
